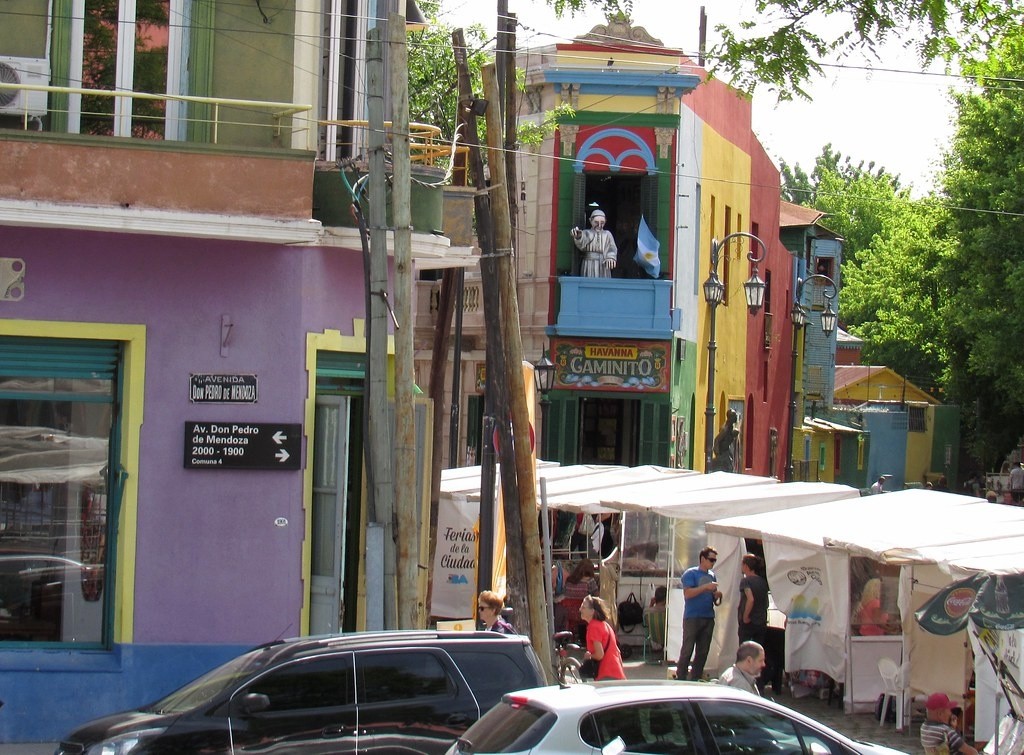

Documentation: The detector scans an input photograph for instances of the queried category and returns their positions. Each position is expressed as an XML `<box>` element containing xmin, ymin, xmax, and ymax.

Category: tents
<box><xmin>429</xmin><ymin>460</ymin><xmax>1024</xmax><ymax>755</ymax></box>
<box><xmin>0</xmin><ymin>424</ymin><xmax>109</xmax><ymax>559</ymax></box>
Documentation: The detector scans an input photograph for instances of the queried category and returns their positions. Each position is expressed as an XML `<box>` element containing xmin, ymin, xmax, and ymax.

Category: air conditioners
<box><xmin>0</xmin><ymin>55</ymin><xmax>51</xmax><ymax>116</ymax></box>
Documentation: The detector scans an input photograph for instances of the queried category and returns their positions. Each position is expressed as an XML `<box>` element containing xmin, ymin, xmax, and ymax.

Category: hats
<box><xmin>926</xmin><ymin>693</ymin><xmax>959</xmax><ymax>710</ymax></box>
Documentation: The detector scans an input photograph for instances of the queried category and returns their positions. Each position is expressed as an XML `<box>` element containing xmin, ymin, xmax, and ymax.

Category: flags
<box><xmin>633</xmin><ymin>214</ymin><xmax>660</xmax><ymax>278</ymax></box>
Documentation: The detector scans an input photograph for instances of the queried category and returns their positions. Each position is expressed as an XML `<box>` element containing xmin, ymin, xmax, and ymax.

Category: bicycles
<box><xmin>550</xmin><ymin>631</ymin><xmax>588</xmax><ymax>684</ymax></box>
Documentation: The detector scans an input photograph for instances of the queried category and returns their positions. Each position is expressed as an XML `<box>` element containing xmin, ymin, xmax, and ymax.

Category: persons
<box><xmin>570</xmin><ymin>512</ymin><xmax>587</xmax><ymax>560</ymax></box>
<box><xmin>478</xmin><ymin>591</ymin><xmax>518</xmax><ymax>635</ymax></box>
<box><xmin>676</xmin><ymin>546</ymin><xmax>722</xmax><ymax>681</ymax></box>
<box><xmin>719</xmin><ymin>641</ymin><xmax>766</xmax><ymax>696</ymax></box>
<box><xmin>713</xmin><ymin>409</ymin><xmax>740</xmax><ymax>469</ymax></box>
<box><xmin>570</xmin><ymin>210</ymin><xmax>617</xmax><ymax>278</ymax></box>
<box><xmin>565</xmin><ymin>559</ymin><xmax>598</xmax><ymax>643</ymax></box>
<box><xmin>580</xmin><ymin>595</ymin><xmax>625</xmax><ymax>680</ymax></box>
<box><xmin>948</xmin><ymin>708</ymin><xmax>961</xmax><ymax>729</ymax></box>
<box><xmin>920</xmin><ymin>692</ymin><xmax>979</xmax><ymax>755</ymax></box>
<box><xmin>737</xmin><ymin>553</ymin><xmax>769</xmax><ymax>695</ymax></box>
<box><xmin>595</xmin><ymin>513</ymin><xmax>613</xmax><ymax>557</ymax></box>
<box><xmin>648</xmin><ymin>586</ymin><xmax>666</xmax><ymax>609</ymax></box>
<box><xmin>1008</xmin><ymin>462</ymin><xmax>1024</xmax><ymax>501</ymax></box>
<box><xmin>859</xmin><ymin>578</ymin><xmax>888</xmax><ymax>635</ymax></box>
<box><xmin>871</xmin><ymin>476</ymin><xmax>885</xmax><ymax>495</ymax></box>
<box><xmin>926</xmin><ymin>482</ymin><xmax>933</xmax><ymax>489</ymax></box>
<box><xmin>963</xmin><ymin>474</ymin><xmax>979</xmax><ymax>496</ymax></box>
<box><xmin>978</xmin><ymin>473</ymin><xmax>986</xmax><ymax>491</ymax></box>
<box><xmin>986</xmin><ymin>491</ymin><xmax>997</xmax><ymax>503</ymax></box>
<box><xmin>999</xmin><ymin>491</ymin><xmax>1017</xmax><ymax>504</ymax></box>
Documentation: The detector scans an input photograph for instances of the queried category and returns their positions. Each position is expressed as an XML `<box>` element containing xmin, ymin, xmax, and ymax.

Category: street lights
<box><xmin>703</xmin><ymin>230</ymin><xmax>768</xmax><ymax>474</ymax></box>
<box><xmin>783</xmin><ymin>273</ymin><xmax>838</xmax><ymax>478</ymax></box>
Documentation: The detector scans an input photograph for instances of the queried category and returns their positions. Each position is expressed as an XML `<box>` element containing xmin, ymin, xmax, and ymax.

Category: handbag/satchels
<box><xmin>580</xmin><ymin>658</ymin><xmax>600</xmax><ymax>678</ymax></box>
<box><xmin>619</xmin><ymin>593</ymin><xmax>643</xmax><ymax>626</ymax></box>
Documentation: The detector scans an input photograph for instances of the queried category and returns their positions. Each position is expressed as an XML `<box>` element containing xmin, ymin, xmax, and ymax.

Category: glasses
<box><xmin>706</xmin><ymin>557</ymin><xmax>716</xmax><ymax>562</ymax></box>
<box><xmin>477</xmin><ymin>607</ymin><xmax>492</xmax><ymax>610</ymax></box>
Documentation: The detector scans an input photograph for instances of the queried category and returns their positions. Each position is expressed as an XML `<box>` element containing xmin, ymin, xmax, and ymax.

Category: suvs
<box><xmin>49</xmin><ymin>625</ymin><xmax>547</xmax><ymax>755</ymax></box>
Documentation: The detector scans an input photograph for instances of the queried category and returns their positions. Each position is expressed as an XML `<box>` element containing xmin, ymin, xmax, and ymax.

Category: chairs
<box><xmin>649</xmin><ymin>709</ymin><xmax>683</xmax><ymax>751</ymax></box>
<box><xmin>876</xmin><ymin>657</ymin><xmax>909</xmax><ymax>729</ymax></box>
<box><xmin>562</xmin><ymin>595</ymin><xmax>590</xmax><ymax>639</ymax></box>
<box><xmin>643</xmin><ymin>606</ymin><xmax>665</xmax><ymax>665</ymax></box>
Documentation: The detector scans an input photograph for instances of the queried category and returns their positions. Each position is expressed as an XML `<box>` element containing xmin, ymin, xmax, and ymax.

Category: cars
<box><xmin>0</xmin><ymin>544</ymin><xmax>106</xmax><ymax>639</ymax></box>
<box><xmin>443</xmin><ymin>679</ymin><xmax>907</xmax><ymax>755</ymax></box>
